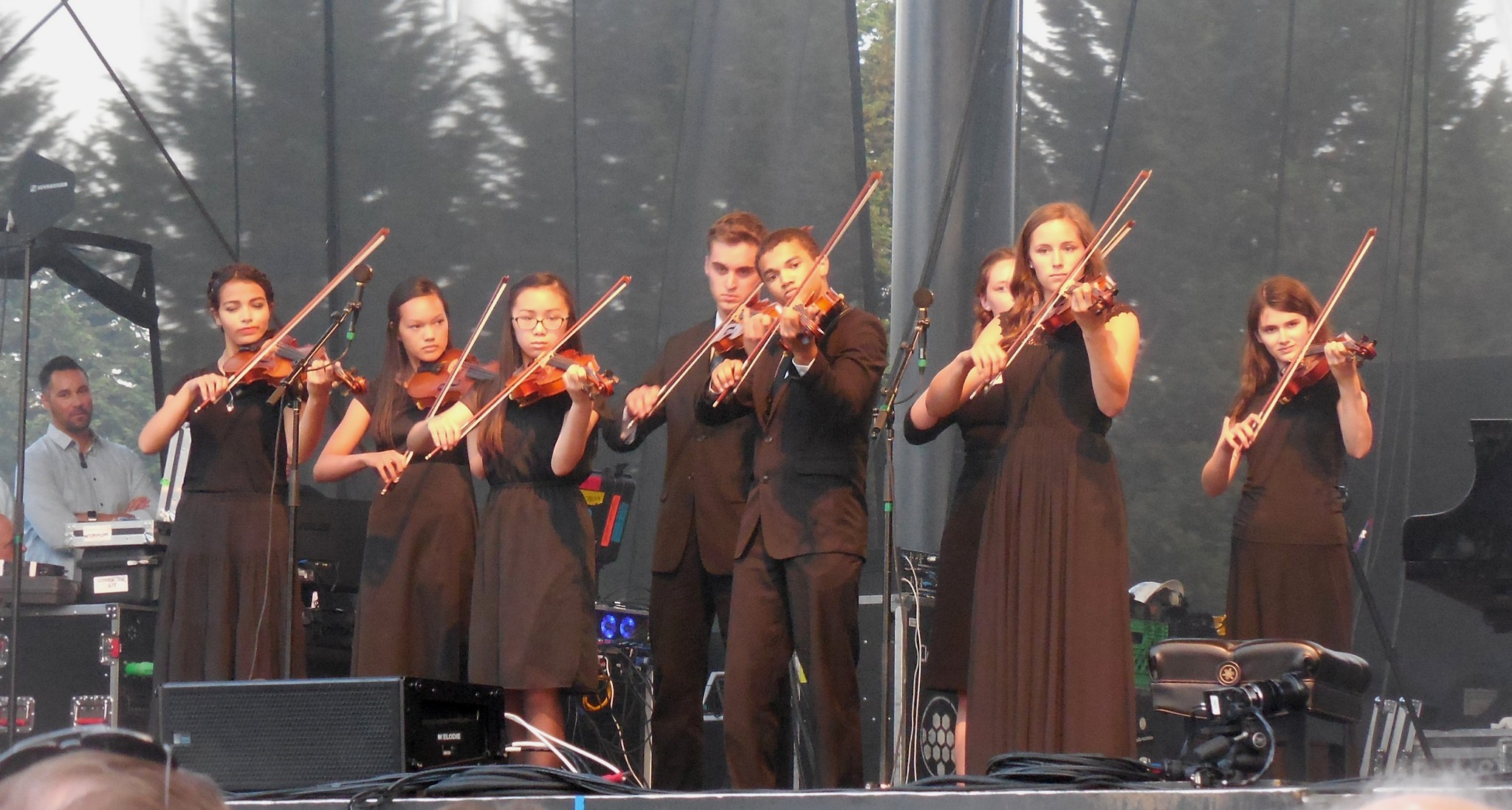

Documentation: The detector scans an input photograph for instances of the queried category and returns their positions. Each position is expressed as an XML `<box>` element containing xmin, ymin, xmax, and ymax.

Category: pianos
<box><xmin>1402</xmin><ymin>419</ymin><xmax>1511</xmax><ymax>635</ymax></box>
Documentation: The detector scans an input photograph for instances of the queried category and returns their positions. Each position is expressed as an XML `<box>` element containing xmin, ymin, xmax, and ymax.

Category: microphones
<box><xmin>912</xmin><ymin>287</ymin><xmax>935</xmax><ymax>376</ymax></box>
<box><xmin>347</xmin><ymin>264</ymin><xmax>373</xmax><ymax>339</ymax></box>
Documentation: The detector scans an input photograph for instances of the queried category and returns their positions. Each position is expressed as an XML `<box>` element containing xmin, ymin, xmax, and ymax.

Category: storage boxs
<box><xmin>74</xmin><ymin>544</ymin><xmax>167</xmax><ymax>605</ymax></box>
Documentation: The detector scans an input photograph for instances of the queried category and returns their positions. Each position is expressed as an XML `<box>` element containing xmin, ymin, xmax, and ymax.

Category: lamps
<box><xmin>1162</xmin><ymin>670</ymin><xmax>1310</xmax><ymax>789</ymax></box>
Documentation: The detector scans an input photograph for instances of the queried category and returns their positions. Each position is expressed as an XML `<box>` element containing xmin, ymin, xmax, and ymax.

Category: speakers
<box><xmin>151</xmin><ymin>676</ymin><xmax>507</xmax><ymax>797</ymax></box>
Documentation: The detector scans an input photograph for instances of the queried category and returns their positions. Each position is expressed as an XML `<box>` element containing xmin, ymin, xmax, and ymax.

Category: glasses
<box><xmin>510</xmin><ymin>315</ymin><xmax>569</xmax><ymax>331</ymax></box>
<box><xmin>1</xmin><ymin>725</ymin><xmax>179</xmax><ymax>809</ymax></box>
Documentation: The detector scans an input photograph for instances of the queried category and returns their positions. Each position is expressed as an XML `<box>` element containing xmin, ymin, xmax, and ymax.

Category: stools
<box><xmin>1147</xmin><ymin>637</ymin><xmax>1374</xmax><ymax>788</ymax></box>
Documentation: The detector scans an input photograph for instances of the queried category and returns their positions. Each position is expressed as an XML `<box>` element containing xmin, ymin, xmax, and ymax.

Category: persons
<box><xmin>603</xmin><ymin>208</ymin><xmax>777</xmax><ymax>792</ymax></box>
<box><xmin>312</xmin><ymin>278</ymin><xmax>479</xmax><ymax>686</ymax></box>
<box><xmin>694</xmin><ymin>229</ymin><xmax>888</xmax><ymax>787</ymax></box>
<box><xmin>138</xmin><ymin>261</ymin><xmax>334</xmax><ymax>678</ymax></box>
<box><xmin>924</xmin><ymin>201</ymin><xmax>1142</xmax><ymax>776</ymax></box>
<box><xmin>1</xmin><ymin>478</ymin><xmax>25</xmax><ymax>570</ymax></box>
<box><xmin>1202</xmin><ymin>276</ymin><xmax>1375</xmax><ymax>648</ymax></box>
<box><xmin>0</xmin><ymin>720</ymin><xmax>229</xmax><ymax>810</ymax></box>
<box><xmin>19</xmin><ymin>355</ymin><xmax>161</xmax><ymax>593</ymax></box>
<box><xmin>904</xmin><ymin>249</ymin><xmax>1022</xmax><ymax>784</ymax></box>
<box><xmin>406</xmin><ymin>271</ymin><xmax>617</xmax><ymax>775</ymax></box>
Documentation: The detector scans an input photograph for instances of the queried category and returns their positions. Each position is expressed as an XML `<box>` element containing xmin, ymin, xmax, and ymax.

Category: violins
<box><xmin>1276</xmin><ymin>332</ymin><xmax>1378</xmax><ymax>406</ymax></box>
<box><xmin>778</xmin><ymin>287</ymin><xmax>844</xmax><ymax>353</ymax></box>
<box><xmin>223</xmin><ymin>332</ymin><xmax>369</xmax><ymax>399</ymax></box>
<box><xmin>1039</xmin><ymin>274</ymin><xmax>1119</xmax><ymax>334</ymax></box>
<box><xmin>505</xmin><ymin>350</ymin><xmax>620</xmax><ymax>407</ymax></box>
<box><xmin>711</xmin><ymin>297</ymin><xmax>782</xmax><ymax>354</ymax></box>
<box><xmin>407</xmin><ymin>347</ymin><xmax>501</xmax><ymax>411</ymax></box>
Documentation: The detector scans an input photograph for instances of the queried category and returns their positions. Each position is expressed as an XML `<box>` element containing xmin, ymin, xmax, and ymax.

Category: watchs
<box><xmin>87</xmin><ymin>510</ymin><xmax>97</xmax><ymax>522</ymax></box>
